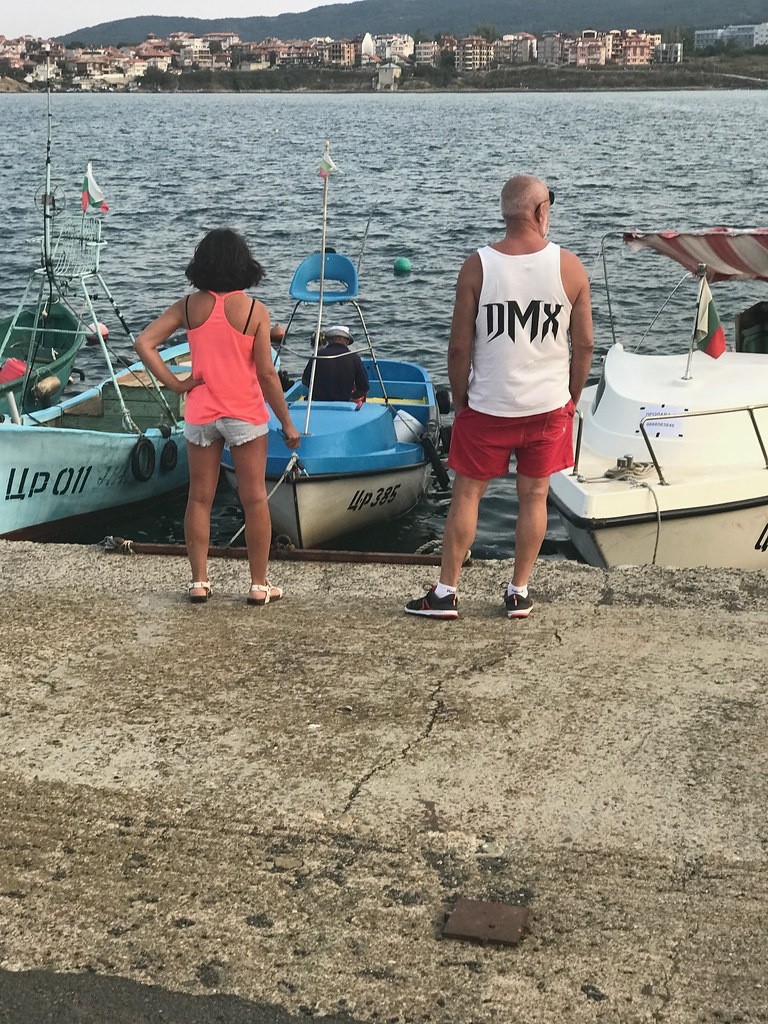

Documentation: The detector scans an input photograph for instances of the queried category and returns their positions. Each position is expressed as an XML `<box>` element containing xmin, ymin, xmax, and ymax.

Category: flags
<box><xmin>318</xmin><ymin>151</ymin><xmax>342</xmax><ymax>181</ymax></box>
<box><xmin>80</xmin><ymin>162</ymin><xmax>109</xmax><ymax>216</ymax></box>
<box><xmin>691</xmin><ymin>275</ymin><xmax>726</xmax><ymax>358</ymax></box>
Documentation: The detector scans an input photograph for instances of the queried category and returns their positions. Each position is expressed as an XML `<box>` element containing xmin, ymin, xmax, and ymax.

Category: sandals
<box><xmin>246</xmin><ymin>578</ymin><xmax>283</xmax><ymax>606</ymax></box>
<box><xmin>187</xmin><ymin>578</ymin><xmax>213</xmax><ymax>602</ymax></box>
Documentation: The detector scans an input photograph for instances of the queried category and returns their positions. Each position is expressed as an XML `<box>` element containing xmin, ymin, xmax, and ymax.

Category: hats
<box><xmin>325</xmin><ymin>325</ymin><xmax>355</xmax><ymax>345</ymax></box>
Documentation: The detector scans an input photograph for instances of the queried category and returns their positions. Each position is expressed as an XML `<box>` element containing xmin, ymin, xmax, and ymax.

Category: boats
<box><xmin>1</xmin><ymin>43</ymin><xmax>280</xmax><ymax>541</ymax></box>
<box><xmin>548</xmin><ymin>225</ymin><xmax>767</xmax><ymax>567</ymax></box>
<box><xmin>1</xmin><ymin>295</ymin><xmax>84</xmax><ymax>415</ymax></box>
<box><xmin>220</xmin><ymin>140</ymin><xmax>453</xmax><ymax>549</ymax></box>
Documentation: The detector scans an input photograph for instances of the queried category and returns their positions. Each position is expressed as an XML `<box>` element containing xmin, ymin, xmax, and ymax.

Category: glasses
<box><xmin>534</xmin><ymin>190</ymin><xmax>555</xmax><ymax>213</ymax></box>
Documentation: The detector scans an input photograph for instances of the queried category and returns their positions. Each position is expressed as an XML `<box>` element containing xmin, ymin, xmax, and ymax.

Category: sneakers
<box><xmin>501</xmin><ymin>581</ymin><xmax>534</xmax><ymax>619</ymax></box>
<box><xmin>404</xmin><ymin>583</ymin><xmax>459</xmax><ymax>619</ymax></box>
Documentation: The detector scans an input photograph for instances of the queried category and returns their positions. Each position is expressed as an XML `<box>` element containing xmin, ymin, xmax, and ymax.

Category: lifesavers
<box><xmin>437</xmin><ymin>389</ymin><xmax>451</xmax><ymax>415</ymax></box>
<box><xmin>421</xmin><ymin>438</ymin><xmax>451</xmax><ymax>487</ymax></box>
<box><xmin>131</xmin><ymin>439</ymin><xmax>156</xmax><ymax>482</ymax></box>
<box><xmin>440</xmin><ymin>425</ymin><xmax>452</xmax><ymax>453</ymax></box>
<box><xmin>161</xmin><ymin>440</ymin><xmax>177</xmax><ymax>471</ymax></box>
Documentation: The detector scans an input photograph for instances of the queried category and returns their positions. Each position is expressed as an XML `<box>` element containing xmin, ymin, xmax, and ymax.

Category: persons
<box><xmin>134</xmin><ymin>227</ymin><xmax>301</xmax><ymax>606</ymax></box>
<box><xmin>405</xmin><ymin>178</ymin><xmax>593</xmax><ymax>617</ymax></box>
<box><xmin>302</xmin><ymin>326</ymin><xmax>370</xmax><ymax>404</ymax></box>
